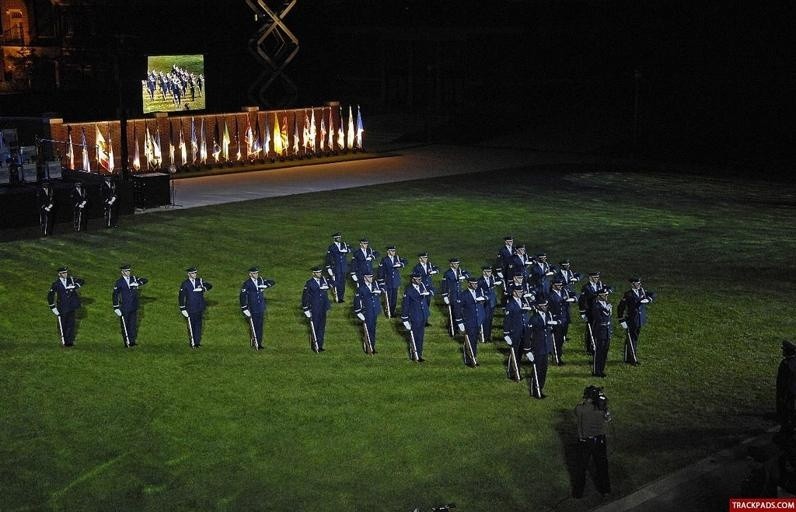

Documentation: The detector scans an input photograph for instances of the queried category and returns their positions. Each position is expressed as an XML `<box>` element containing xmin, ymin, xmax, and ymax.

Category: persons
<box><xmin>178</xmin><ymin>267</ymin><xmax>212</xmax><ymax>350</ymax></box>
<box><xmin>70</xmin><ymin>182</ymin><xmax>89</xmax><ymax>233</ymax></box>
<box><xmin>35</xmin><ymin>153</ymin><xmax>49</xmax><ymax>182</ymax></box>
<box><xmin>100</xmin><ymin>176</ymin><xmax>119</xmax><ymax>228</ymax></box>
<box><xmin>571</xmin><ymin>385</ymin><xmax>611</xmax><ymax>498</ymax></box>
<box><xmin>47</xmin><ymin>267</ymin><xmax>85</xmax><ymax>347</ymax></box>
<box><xmin>112</xmin><ymin>264</ymin><xmax>148</xmax><ymax>348</ymax></box>
<box><xmin>240</xmin><ymin>266</ymin><xmax>275</xmax><ymax>352</ymax></box>
<box><xmin>36</xmin><ymin>179</ymin><xmax>57</xmax><ymax>240</ymax></box>
<box><xmin>6</xmin><ymin>152</ymin><xmax>23</xmax><ymax>185</ymax></box>
<box><xmin>772</xmin><ymin>339</ymin><xmax>795</xmax><ymax>446</ymax></box>
<box><xmin>144</xmin><ymin>63</ymin><xmax>205</xmax><ymax>105</ymax></box>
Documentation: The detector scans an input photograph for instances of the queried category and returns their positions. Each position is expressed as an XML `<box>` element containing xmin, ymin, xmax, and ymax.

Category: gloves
<box><xmin>640</xmin><ymin>298</ymin><xmax>650</xmax><ymax>303</ymax></box>
<box><xmin>403</xmin><ymin>321</ymin><xmax>411</xmax><ymax>330</ymax></box>
<box><xmin>340</xmin><ymin>249</ymin><xmax>347</xmax><ymax>252</ymax></box>
<box><xmin>393</xmin><ymin>264</ymin><xmax>401</xmax><ymax>268</ymax></box>
<box><xmin>304</xmin><ymin>310</ymin><xmax>311</xmax><ymax>317</ymax></box>
<box><xmin>430</xmin><ymin>270</ymin><xmax>437</xmax><ymax>274</ymax></box>
<box><xmin>458</xmin><ymin>323</ymin><xmax>465</xmax><ymax>332</ymax></box>
<box><xmin>328</xmin><ymin>268</ymin><xmax>333</xmax><ymax>276</ymax></box>
<box><xmin>366</xmin><ymin>257</ymin><xmax>372</xmax><ymax>261</ymax></box>
<box><xmin>621</xmin><ymin>321</ymin><xmax>628</xmax><ymax>329</ymax></box>
<box><xmin>357</xmin><ymin>312</ymin><xmax>366</xmax><ymax>321</ymax></box>
<box><xmin>66</xmin><ymin>285</ymin><xmax>75</xmax><ymax>289</ymax></box>
<box><xmin>526</xmin><ymin>351</ymin><xmax>535</xmax><ymax>362</ymax></box>
<box><xmin>320</xmin><ymin>285</ymin><xmax>328</xmax><ymax>290</ymax></box>
<box><xmin>504</xmin><ymin>336</ymin><xmax>512</xmax><ymax>345</ymax></box>
<box><xmin>45</xmin><ymin>204</ymin><xmax>53</xmax><ymax>212</ymax></box>
<box><xmin>182</xmin><ymin>310</ymin><xmax>189</xmax><ymax>317</ymax></box>
<box><xmin>79</xmin><ymin>201</ymin><xmax>87</xmax><ymax>208</ymax></box>
<box><xmin>547</xmin><ymin>320</ymin><xmax>558</xmax><ymax>325</ymax></box>
<box><xmin>522</xmin><ymin>306</ymin><xmax>531</xmax><ymax>311</ymax></box>
<box><xmin>108</xmin><ymin>197</ymin><xmax>116</xmax><ymax>205</ymax></box>
<box><xmin>193</xmin><ymin>288</ymin><xmax>202</xmax><ymax>292</ymax></box>
<box><xmin>243</xmin><ymin>309</ymin><xmax>252</xmax><ymax>316</ymax></box>
<box><xmin>420</xmin><ymin>291</ymin><xmax>429</xmax><ymax>296</ymax></box>
<box><xmin>258</xmin><ymin>284</ymin><xmax>267</xmax><ymax>289</ymax></box>
<box><xmin>372</xmin><ymin>290</ymin><xmax>381</xmax><ymax>294</ymax></box>
<box><xmin>53</xmin><ymin>307</ymin><xmax>59</xmax><ymax>315</ymax></box>
<box><xmin>115</xmin><ymin>308</ymin><xmax>122</xmax><ymax>316</ymax></box>
<box><xmin>129</xmin><ymin>282</ymin><xmax>138</xmax><ymax>287</ymax></box>
<box><xmin>352</xmin><ymin>274</ymin><xmax>358</xmax><ymax>281</ymax></box>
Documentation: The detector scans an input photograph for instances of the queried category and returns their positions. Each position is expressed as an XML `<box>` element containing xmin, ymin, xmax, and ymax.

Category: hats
<box><xmin>247</xmin><ymin>266</ymin><xmax>259</xmax><ymax>273</ymax></box>
<box><xmin>386</xmin><ymin>244</ymin><xmax>396</xmax><ymax>251</ymax></box>
<box><xmin>310</xmin><ymin>265</ymin><xmax>323</xmax><ymax>273</ymax></box>
<box><xmin>448</xmin><ymin>236</ymin><xmax>642</xmax><ymax>306</ymax></box>
<box><xmin>358</xmin><ymin>239</ymin><xmax>369</xmax><ymax>244</ymax></box>
<box><xmin>120</xmin><ymin>264</ymin><xmax>131</xmax><ymax>271</ymax></box>
<box><xmin>57</xmin><ymin>266</ymin><xmax>68</xmax><ymax>273</ymax></box>
<box><xmin>363</xmin><ymin>272</ymin><xmax>375</xmax><ymax>278</ymax></box>
<box><xmin>331</xmin><ymin>232</ymin><xmax>341</xmax><ymax>239</ymax></box>
<box><xmin>184</xmin><ymin>267</ymin><xmax>197</xmax><ymax>273</ymax></box>
<box><xmin>416</xmin><ymin>251</ymin><xmax>428</xmax><ymax>258</ymax></box>
<box><xmin>409</xmin><ymin>272</ymin><xmax>423</xmax><ymax>280</ymax></box>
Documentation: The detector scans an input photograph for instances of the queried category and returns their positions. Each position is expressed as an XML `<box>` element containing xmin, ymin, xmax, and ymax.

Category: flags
<box><xmin>65</xmin><ymin>105</ymin><xmax>365</xmax><ymax>173</ymax></box>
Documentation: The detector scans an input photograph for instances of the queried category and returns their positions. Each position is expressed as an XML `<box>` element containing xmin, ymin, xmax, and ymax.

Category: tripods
<box><xmin>168</xmin><ymin>173</ymin><xmax>183</xmax><ymax>207</ymax></box>
